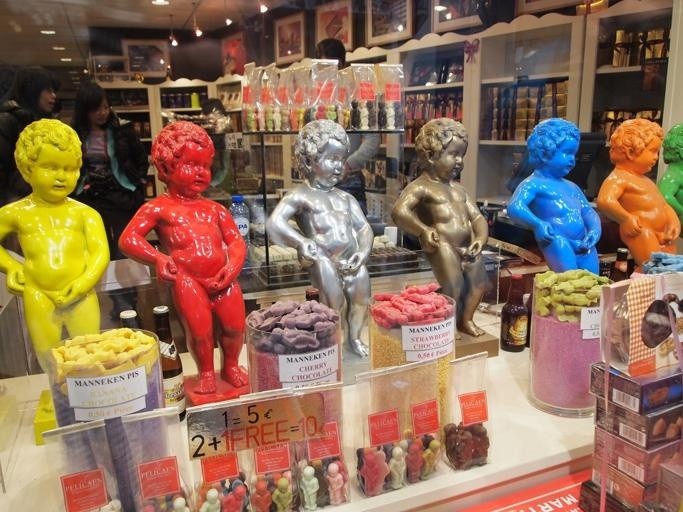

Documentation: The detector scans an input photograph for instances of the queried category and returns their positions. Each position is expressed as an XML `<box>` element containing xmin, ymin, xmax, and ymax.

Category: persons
<box><xmin>118</xmin><ymin>121</ymin><xmax>250</xmax><ymax>395</ymax></box>
<box><xmin>67</xmin><ymin>81</ymin><xmax>149</xmax><ymax>330</ymax></box>
<box><xmin>0</xmin><ymin>63</ymin><xmax>61</xmax><ymax>256</ymax></box>
<box><xmin>658</xmin><ymin>122</ymin><xmax>683</xmax><ymax>237</ymax></box>
<box><xmin>506</xmin><ymin>117</ymin><xmax>602</xmax><ymax>276</ymax></box>
<box><xmin>0</xmin><ymin>118</ymin><xmax>110</xmax><ymax>414</ymax></box>
<box><xmin>597</xmin><ymin>118</ymin><xmax>681</xmax><ymax>273</ymax></box>
<box><xmin>314</xmin><ymin>38</ymin><xmax>380</xmax><ymax>217</ymax></box>
<box><xmin>266</xmin><ymin>119</ymin><xmax>374</xmax><ymax>359</ymax></box>
<box><xmin>391</xmin><ymin>117</ymin><xmax>489</xmax><ymax>339</ymax></box>
<box><xmin>200</xmin><ymin>98</ymin><xmax>226</xmax><ymax>121</ymax></box>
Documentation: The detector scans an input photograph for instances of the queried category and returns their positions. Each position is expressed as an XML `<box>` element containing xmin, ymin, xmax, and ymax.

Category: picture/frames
<box><xmin>219</xmin><ymin>30</ymin><xmax>248</xmax><ymax>76</ymax></box>
<box><xmin>120</xmin><ymin>38</ymin><xmax>171</xmax><ymax>79</ymax></box>
<box><xmin>91</xmin><ymin>55</ymin><xmax>130</xmax><ymax>83</ymax></box>
<box><xmin>273</xmin><ymin>10</ymin><xmax>306</xmax><ymax>68</ymax></box>
<box><xmin>364</xmin><ymin>0</ymin><xmax>414</xmax><ymax>48</ymax></box>
<box><xmin>313</xmin><ymin>0</ymin><xmax>356</xmax><ymax>52</ymax></box>
<box><xmin>426</xmin><ymin>0</ymin><xmax>483</xmax><ymax>35</ymax></box>
<box><xmin>512</xmin><ymin>0</ymin><xmax>582</xmax><ymax>18</ymax></box>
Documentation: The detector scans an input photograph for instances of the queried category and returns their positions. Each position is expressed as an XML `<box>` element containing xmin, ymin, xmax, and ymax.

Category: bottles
<box><xmin>499</xmin><ymin>275</ymin><xmax>529</xmax><ymax>352</ymax></box>
<box><xmin>182</xmin><ymin>94</ymin><xmax>191</xmax><ymax>109</ymax></box>
<box><xmin>173</xmin><ymin>96</ymin><xmax>181</xmax><ymax>108</ymax></box>
<box><xmin>121</xmin><ymin>311</ymin><xmax>138</xmax><ymax>328</ymax></box>
<box><xmin>228</xmin><ymin>196</ymin><xmax>251</xmax><ymax>268</ymax></box>
<box><xmin>154</xmin><ymin>307</ymin><xmax>185</xmax><ymax>421</ymax></box>
<box><xmin>143</xmin><ymin>123</ymin><xmax>149</xmax><ymax>135</ymax></box>
<box><xmin>162</xmin><ymin>94</ymin><xmax>170</xmax><ymax>111</ymax></box>
<box><xmin>192</xmin><ymin>91</ymin><xmax>203</xmax><ymax>111</ymax></box>
<box><xmin>198</xmin><ymin>92</ymin><xmax>209</xmax><ymax>109</ymax></box>
<box><xmin>302</xmin><ymin>288</ymin><xmax>319</xmax><ymax>301</ymax></box>
<box><xmin>610</xmin><ymin>246</ymin><xmax>631</xmax><ymax>281</ymax></box>
<box><xmin>145</xmin><ymin>175</ymin><xmax>153</xmax><ymax>197</ymax></box>
<box><xmin>134</xmin><ymin>120</ymin><xmax>140</xmax><ymax>137</ymax></box>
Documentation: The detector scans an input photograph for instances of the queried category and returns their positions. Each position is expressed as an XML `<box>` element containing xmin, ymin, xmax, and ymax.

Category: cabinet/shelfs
<box><xmin>0</xmin><ymin>302</ymin><xmax>599</xmax><ymax>512</ymax></box>
<box><xmin>465</xmin><ymin>11</ymin><xmax>586</xmax><ymax>261</ymax></box>
<box><xmin>245</xmin><ymin>66</ymin><xmax>288</xmax><ymax>194</ymax></box>
<box><xmin>344</xmin><ymin>45</ymin><xmax>392</xmax><ymax>199</ymax></box>
<box><xmin>577</xmin><ymin>1</ymin><xmax>681</xmax><ymax>191</ymax></box>
<box><xmin>286</xmin><ymin>134</ymin><xmax>304</xmax><ymax>192</ymax></box>
<box><xmin>212</xmin><ymin>72</ymin><xmax>245</xmax><ymax>136</ymax></box>
<box><xmin>154</xmin><ymin>77</ymin><xmax>212</xmax><ymax>197</ymax></box>
<box><xmin>95</xmin><ymin>80</ymin><xmax>160</xmax><ymax>200</ymax></box>
<box><xmin>390</xmin><ymin>32</ymin><xmax>470</xmax><ymax>199</ymax></box>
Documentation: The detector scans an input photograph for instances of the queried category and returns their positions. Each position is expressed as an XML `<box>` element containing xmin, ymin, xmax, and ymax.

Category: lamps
<box><xmin>224</xmin><ymin>0</ymin><xmax>232</xmax><ymax>27</ymax></box>
<box><xmin>258</xmin><ymin>0</ymin><xmax>267</xmax><ymax>13</ymax></box>
<box><xmin>169</xmin><ymin>14</ymin><xmax>178</xmax><ymax>46</ymax></box>
<box><xmin>192</xmin><ymin>2</ymin><xmax>202</xmax><ymax>37</ymax></box>
